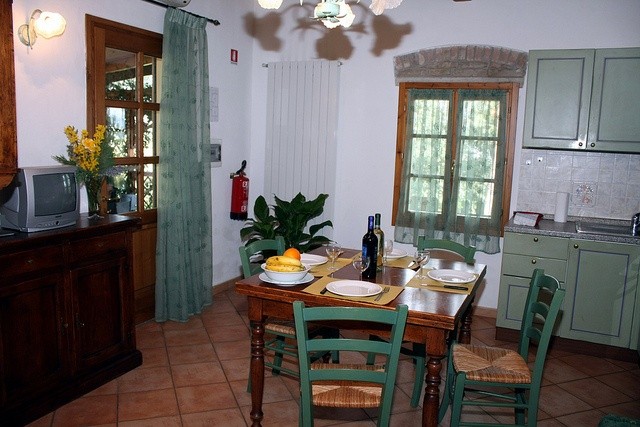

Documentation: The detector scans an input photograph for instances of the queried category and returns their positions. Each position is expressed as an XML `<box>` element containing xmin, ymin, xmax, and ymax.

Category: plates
<box><xmin>383</xmin><ymin>248</ymin><xmax>408</xmax><ymax>261</ymax></box>
<box><xmin>428</xmin><ymin>269</ymin><xmax>476</xmax><ymax>285</ymax></box>
<box><xmin>301</xmin><ymin>253</ymin><xmax>328</xmax><ymax>267</ymax></box>
<box><xmin>259</xmin><ymin>273</ymin><xmax>314</xmax><ymax>287</ymax></box>
<box><xmin>326</xmin><ymin>280</ymin><xmax>383</xmax><ymax>298</ymax></box>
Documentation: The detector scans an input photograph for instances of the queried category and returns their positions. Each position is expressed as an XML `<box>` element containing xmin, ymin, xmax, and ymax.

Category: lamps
<box><xmin>19</xmin><ymin>8</ymin><xmax>67</xmax><ymax>50</ymax></box>
<box><xmin>257</xmin><ymin>0</ymin><xmax>402</xmax><ymax>28</ymax></box>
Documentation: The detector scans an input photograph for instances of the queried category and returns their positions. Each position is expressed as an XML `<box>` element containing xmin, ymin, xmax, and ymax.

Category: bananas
<box><xmin>266</xmin><ymin>256</ymin><xmax>306</xmax><ymax>272</ymax></box>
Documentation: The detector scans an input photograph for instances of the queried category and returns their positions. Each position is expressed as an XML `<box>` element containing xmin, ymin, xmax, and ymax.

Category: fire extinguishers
<box><xmin>230</xmin><ymin>159</ymin><xmax>249</xmax><ymax>221</ymax></box>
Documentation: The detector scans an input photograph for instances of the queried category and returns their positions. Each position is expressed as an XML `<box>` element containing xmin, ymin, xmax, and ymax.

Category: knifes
<box><xmin>421</xmin><ymin>283</ymin><xmax>469</xmax><ymax>291</ymax></box>
<box><xmin>408</xmin><ymin>257</ymin><xmax>421</xmax><ymax>268</ymax></box>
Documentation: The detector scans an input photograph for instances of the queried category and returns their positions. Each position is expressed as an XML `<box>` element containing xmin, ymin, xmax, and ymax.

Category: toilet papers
<box><xmin>554</xmin><ymin>192</ymin><xmax>569</xmax><ymax>223</ymax></box>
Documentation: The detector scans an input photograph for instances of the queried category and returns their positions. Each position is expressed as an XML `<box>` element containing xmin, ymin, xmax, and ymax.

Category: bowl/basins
<box><xmin>261</xmin><ymin>263</ymin><xmax>308</xmax><ymax>282</ymax></box>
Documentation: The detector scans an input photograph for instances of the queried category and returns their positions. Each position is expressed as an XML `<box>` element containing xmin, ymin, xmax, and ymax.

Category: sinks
<box><xmin>575</xmin><ymin>220</ymin><xmax>635</xmax><ymax>238</ymax></box>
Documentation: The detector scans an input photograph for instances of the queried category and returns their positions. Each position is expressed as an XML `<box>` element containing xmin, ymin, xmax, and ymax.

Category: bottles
<box><xmin>361</xmin><ymin>216</ymin><xmax>378</xmax><ymax>283</ymax></box>
<box><xmin>374</xmin><ymin>213</ymin><xmax>385</xmax><ymax>272</ymax></box>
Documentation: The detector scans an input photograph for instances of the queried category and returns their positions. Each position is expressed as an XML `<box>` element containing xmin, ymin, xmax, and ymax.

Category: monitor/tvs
<box><xmin>0</xmin><ymin>165</ymin><xmax>80</xmax><ymax>232</ymax></box>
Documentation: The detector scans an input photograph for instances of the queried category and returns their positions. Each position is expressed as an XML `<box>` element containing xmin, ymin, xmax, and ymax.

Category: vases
<box><xmin>85</xmin><ymin>179</ymin><xmax>101</xmax><ymax>212</ymax></box>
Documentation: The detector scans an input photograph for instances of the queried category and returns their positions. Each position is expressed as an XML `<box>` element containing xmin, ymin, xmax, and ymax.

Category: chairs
<box><xmin>367</xmin><ymin>235</ymin><xmax>476</xmax><ymax>407</ymax></box>
<box><xmin>437</xmin><ymin>268</ymin><xmax>566</xmax><ymax>426</ymax></box>
<box><xmin>239</xmin><ymin>234</ymin><xmax>340</xmax><ymax>393</ymax></box>
<box><xmin>292</xmin><ymin>299</ymin><xmax>408</xmax><ymax>425</ymax></box>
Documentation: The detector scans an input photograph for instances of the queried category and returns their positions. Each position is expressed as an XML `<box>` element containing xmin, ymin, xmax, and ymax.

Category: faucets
<box><xmin>632</xmin><ymin>213</ymin><xmax>640</xmax><ymax>234</ymax></box>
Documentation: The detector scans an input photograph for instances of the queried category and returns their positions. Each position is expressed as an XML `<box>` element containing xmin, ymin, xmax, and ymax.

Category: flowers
<box><xmin>52</xmin><ymin>123</ymin><xmax>124</xmax><ymax>183</ymax></box>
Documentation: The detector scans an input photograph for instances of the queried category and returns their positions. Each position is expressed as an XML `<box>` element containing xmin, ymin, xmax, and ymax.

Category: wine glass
<box><xmin>415</xmin><ymin>250</ymin><xmax>431</xmax><ymax>280</ymax></box>
<box><xmin>383</xmin><ymin>239</ymin><xmax>394</xmax><ymax>265</ymax></box>
<box><xmin>352</xmin><ymin>256</ymin><xmax>370</xmax><ymax>280</ymax></box>
<box><xmin>326</xmin><ymin>241</ymin><xmax>342</xmax><ymax>271</ymax></box>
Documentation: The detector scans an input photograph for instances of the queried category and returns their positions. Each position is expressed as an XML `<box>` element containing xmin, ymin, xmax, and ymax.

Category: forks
<box><xmin>375</xmin><ymin>286</ymin><xmax>390</xmax><ymax>301</ymax></box>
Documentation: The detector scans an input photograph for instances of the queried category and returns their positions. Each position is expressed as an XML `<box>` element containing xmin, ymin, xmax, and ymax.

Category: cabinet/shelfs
<box><xmin>0</xmin><ymin>212</ymin><xmax>143</xmax><ymax>426</ymax></box>
<box><xmin>495</xmin><ymin>233</ymin><xmax>570</xmax><ymax>339</ymax></box>
<box><xmin>522</xmin><ymin>47</ymin><xmax>640</xmax><ymax>154</ymax></box>
<box><xmin>560</xmin><ymin>240</ymin><xmax>640</xmax><ymax>352</ymax></box>
